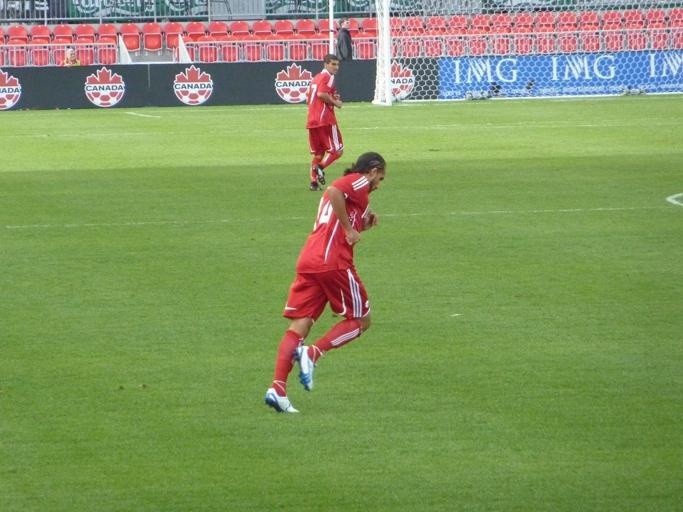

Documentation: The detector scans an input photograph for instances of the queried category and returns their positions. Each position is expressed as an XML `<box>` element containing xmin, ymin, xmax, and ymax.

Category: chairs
<box><xmin>0</xmin><ymin>8</ymin><xmax>683</xmax><ymax>70</ymax></box>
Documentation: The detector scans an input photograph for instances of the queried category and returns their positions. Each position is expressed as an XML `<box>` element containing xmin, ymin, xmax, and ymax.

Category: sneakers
<box><xmin>309</xmin><ymin>163</ymin><xmax>325</xmax><ymax>191</ymax></box>
<box><xmin>264</xmin><ymin>387</ymin><xmax>300</xmax><ymax>413</ymax></box>
<box><xmin>294</xmin><ymin>345</ymin><xmax>314</xmax><ymax>391</ymax></box>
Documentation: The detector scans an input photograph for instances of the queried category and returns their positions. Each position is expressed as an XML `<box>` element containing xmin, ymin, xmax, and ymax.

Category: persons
<box><xmin>261</xmin><ymin>149</ymin><xmax>388</xmax><ymax>414</ymax></box>
<box><xmin>303</xmin><ymin>53</ymin><xmax>344</xmax><ymax>191</ymax></box>
<box><xmin>336</xmin><ymin>16</ymin><xmax>353</xmax><ymax>61</ymax></box>
